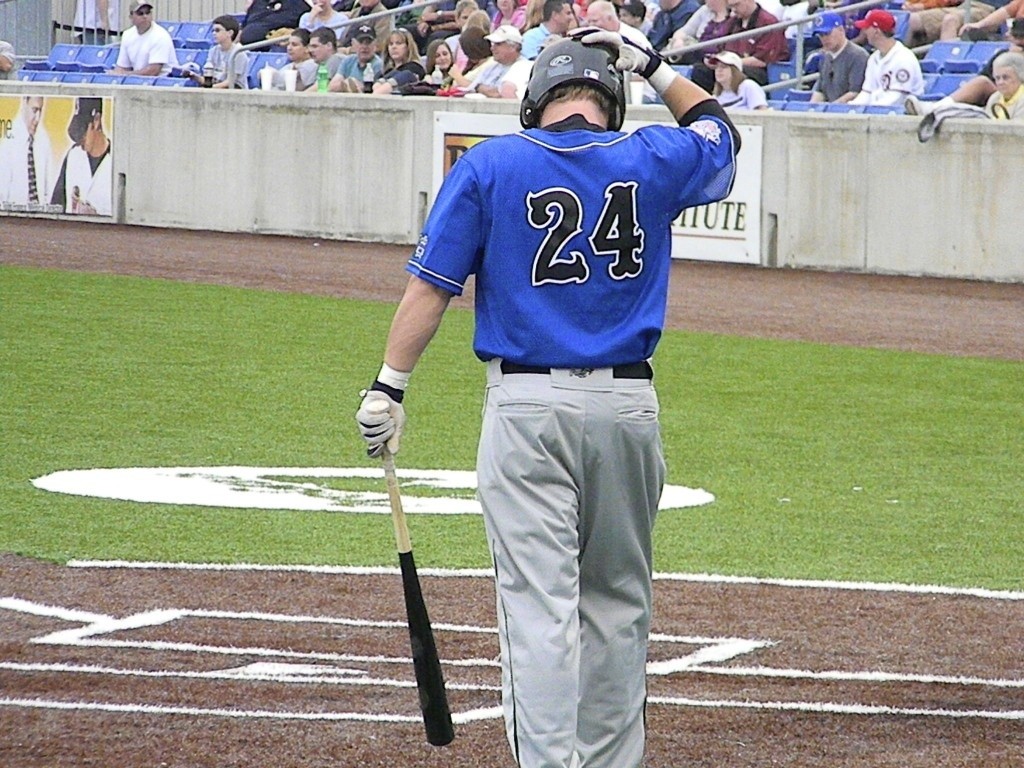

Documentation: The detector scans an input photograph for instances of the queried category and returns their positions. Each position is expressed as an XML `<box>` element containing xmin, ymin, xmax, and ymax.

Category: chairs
<box><xmin>15</xmin><ymin>14</ymin><xmax>292</xmax><ymax>88</ymax></box>
<box><xmin>642</xmin><ymin>0</ymin><xmax>1010</xmax><ymax>117</ymax></box>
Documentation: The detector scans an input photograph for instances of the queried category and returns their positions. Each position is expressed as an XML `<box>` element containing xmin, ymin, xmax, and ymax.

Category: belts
<box><xmin>500</xmin><ymin>359</ymin><xmax>653</xmax><ymax>380</ymax></box>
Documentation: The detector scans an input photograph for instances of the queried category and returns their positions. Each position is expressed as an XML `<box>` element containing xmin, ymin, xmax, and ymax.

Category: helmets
<box><xmin>520</xmin><ymin>36</ymin><xmax>627</xmax><ymax>132</ymax></box>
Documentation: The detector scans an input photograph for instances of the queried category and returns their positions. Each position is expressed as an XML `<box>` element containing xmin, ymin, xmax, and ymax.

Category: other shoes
<box><xmin>905</xmin><ymin>94</ymin><xmax>929</xmax><ymax>116</ymax></box>
<box><xmin>342</xmin><ymin>77</ymin><xmax>362</xmax><ymax>93</ymax></box>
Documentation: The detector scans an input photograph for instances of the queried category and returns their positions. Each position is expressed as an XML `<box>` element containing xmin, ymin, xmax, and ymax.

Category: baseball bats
<box><xmin>366</xmin><ymin>398</ymin><xmax>457</xmax><ymax>746</ymax></box>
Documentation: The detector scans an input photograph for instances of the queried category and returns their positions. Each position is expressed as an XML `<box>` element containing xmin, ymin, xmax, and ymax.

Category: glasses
<box><xmin>390</xmin><ymin>27</ymin><xmax>408</xmax><ymax>43</ymax></box>
<box><xmin>137</xmin><ymin>10</ymin><xmax>151</xmax><ymax>15</ymax></box>
<box><xmin>211</xmin><ymin>28</ymin><xmax>227</xmax><ymax>32</ymax></box>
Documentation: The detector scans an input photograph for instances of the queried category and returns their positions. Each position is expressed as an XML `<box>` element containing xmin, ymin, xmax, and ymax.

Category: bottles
<box><xmin>364</xmin><ymin>63</ymin><xmax>374</xmax><ymax>94</ymax></box>
<box><xmin>318</xmin><ymin>63</ymin><xmax>329</xmax><ymax>92</ymax></box>
<box><xmin>203</xmin><ymin>57</ymin><xmax>213</xmax><ymax>88</ymax></box>
<box><xmin>431</xmin><ymin>65</ymin><xmax>443</xmax><ymax>84</ymax></box>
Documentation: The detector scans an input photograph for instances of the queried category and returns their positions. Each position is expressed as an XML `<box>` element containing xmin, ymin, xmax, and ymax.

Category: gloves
<box><xmin>354</xmin><ymin>362</ymin><xmax>413</xmax><ymax>458</ymax></box>
<box><xmin>567</xmin><ymin>25</ymin><xmax>680</xmax><ymax>95</ymax></box>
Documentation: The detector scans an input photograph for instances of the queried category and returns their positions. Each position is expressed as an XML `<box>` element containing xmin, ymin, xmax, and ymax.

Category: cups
<box><xmin>260</xmin><ymin>69</ymin><xmax>274</xmax><ymax>90</ymax></box>
<box><xmin>629</xmin><ymin>82</ymin><xmax>643</xmax><ymax>104</ymax></box>
<box><xmin>284</xmin><ymin>69</ymin><xmax>300</xmax><ymax>91</ymax></box>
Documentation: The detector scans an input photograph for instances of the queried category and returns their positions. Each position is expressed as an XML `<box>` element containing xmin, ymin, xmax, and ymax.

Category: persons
<box><xmin>182</xmin><ymin>15</ymin><xmax>250</xmax><ymax>89</ymax></box>
<box><xmin>582</xmin><ymin>0</ymin><xmax>1024</xmax><ymax>122</ymax></box>
<box><xmin>355</xmin><ymin>24</ymin><xmax>744</xmax><ymax>766</ymax></box>
<box><xmin>365</xmin><ymin>28</ymin><xmax>425</xmax><ymax>95</ymax></box>
<box><xmin>258</xmin><ymin>28</ymin><xmax>318</xmax><ymax>93</ymax></box>
<box><xmin>303</xmin><ymin>26</ymin><xmax>347</xmax><ymax>92</ymax></box>
<box><xmin>73</xmin><ymin>0</ymin><xmax>118</xmax><ymax>45</ymax></box>
<box><xmin>0</xmin><ymin>40</ymin><xmax>19</xmax><ymax>81</ymax></box>
<box><xmin>332</xmin><ymin>0</ymin><xmax>580</xmax><ymax>99</ymax></box>
<box><xmin>298</xmin><ymin>0</ymin><xmax>351</xmax><ymax>39</ymax></box>
<box><xmin>0</xmin><ymin>95</ymin><xmax>54</xmax><ymax>207</ymax></box>
<box><xmin>51</xmin><ymin>96</ymin><xmax>114</xmax><ymax>215</ymax></box>
<box><xmin>329</xmin><ymin>24</ymin><xmax>385</xmax><ymax>95</ymax></box>
<box><xmin>236</xmin><ymin>0</ymin><xmax>311</xmax><ymax>52</ymax></box>
<box><xmin>107</xmin><ymin>0</ymin><xmax>179</xmax><ymax>79</ymax></box>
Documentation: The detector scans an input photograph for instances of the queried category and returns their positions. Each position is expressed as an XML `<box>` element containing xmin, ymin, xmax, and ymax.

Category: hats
<box><xmin>709</xmin><ymin>50</ymin><xmax>743</xmax><ymax>71</ymax></box>
<box><xmin>67</xmin><ymin>98</ymin><xmax>103</xmax><ymax>142</ymax></box>
<box><xmin>353</xmin><ymin>24</ymin><xmax>376</xmax><ymax>40</ymax></box>
<box><xmin>854</xmin><ymin>9</ymin><xmax>896</xmax><ymax>32</ymax></box>
<box><xmin>483</xmin><ymin>25</ymin><xmax>523</xmax><ymax>45</ymax></box>
<box><xmin>810</xmin><ymin>8</ymin><xmax>844</xmax><ymax>36</ymax></box>
<box><xmin>128</xmin><ymin>0</ymin><xmax>153</xmax><ymax>12</ymax></box>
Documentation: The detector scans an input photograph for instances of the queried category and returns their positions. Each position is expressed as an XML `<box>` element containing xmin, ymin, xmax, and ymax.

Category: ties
<box><xmin>27</xmin><ymin>134</ymin><xmax>39</xmax><ymax>204</ymax></box>
